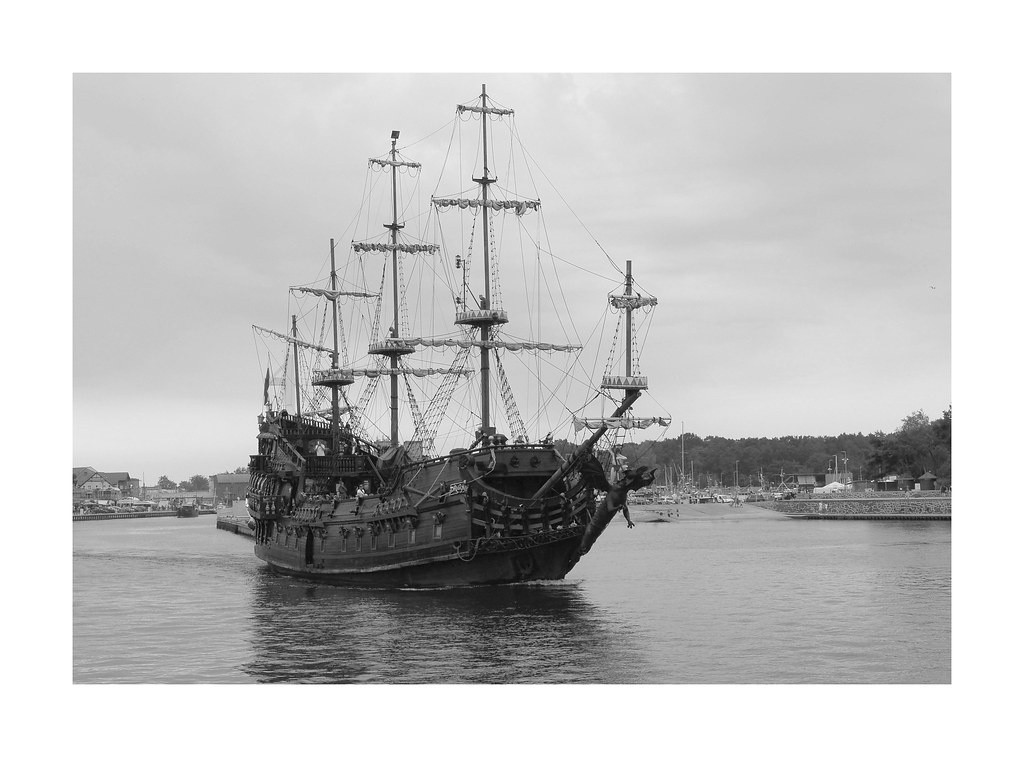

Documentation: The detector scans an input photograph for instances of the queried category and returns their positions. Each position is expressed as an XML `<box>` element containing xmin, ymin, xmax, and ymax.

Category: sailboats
<box><xmin>245</xmin><ymin>84</ymin><xmax>671</xmax><ymax>589</ymax></box>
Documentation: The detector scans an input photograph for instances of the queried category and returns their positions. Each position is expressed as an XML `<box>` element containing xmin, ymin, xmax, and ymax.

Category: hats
<box><xmin>359</xmin><ymin>485</ymin><xmax>363</xmax><ymax>487</ymax></box>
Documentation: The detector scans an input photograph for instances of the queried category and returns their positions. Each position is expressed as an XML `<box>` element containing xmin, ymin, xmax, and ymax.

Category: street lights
<box><xmin>841</xmin><ymin>451</ymin><xmax>846</xmax><ymax>485</ymax></box>
<box><xmin>736</xmin><ymin>460</ymin><xmax>739</xmax><ymax>486</ymax></box>
<box><xmin>833</xmin><ymin>455</ymin><xmax>837</xmax><ymax>481</ymax></box>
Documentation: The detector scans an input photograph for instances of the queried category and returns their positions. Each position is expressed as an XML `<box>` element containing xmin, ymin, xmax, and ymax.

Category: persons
<box><xmin>515</xmin><ymin>435</ymin><xmax>529</xmax><ymax>448</ymax></box>
<box><xmin>338</xmin><ymin>482</ymin><xmax>347</xmax><ymax>498</ymax></box>
<box><xmin>357</xmin><ymin>485</ymin><xmax>365</xmax><ymax>497</ymax></box>
<box><xmin>315</xmin><ymin>440</ymin><xmax>325</xmax><ymax>456</ymax></box>
<box><xmin>377</xmin><ymin>483</ymin><xmax>385</xmax><ymax>495</ymax></box>
<box><xmin>343</xmin><ymin>441</ymin><xmax>352</xmax><ymax>455</ymax></box>
<box><xmin>341</xmin><ymin>422</ymin><xmax>354</xmax><ymax>430</ymax></box>
